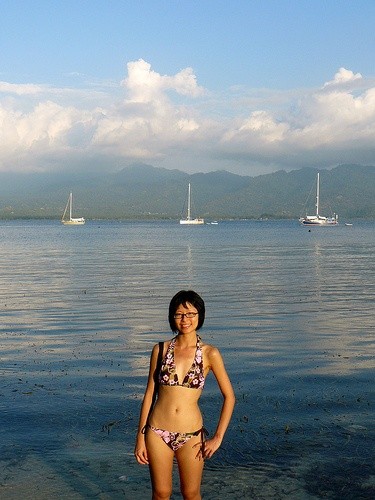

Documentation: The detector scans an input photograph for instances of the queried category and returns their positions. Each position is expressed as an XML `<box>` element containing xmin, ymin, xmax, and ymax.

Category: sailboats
<box><xmin>179</xmin><ymin>181</ymin><xmax>205</xmax><ymax>224</ymax></box>
<box><xmin>61</xmin><ymin>193</ymin><xmax>86</xmax><ymax>225</ymax></box>
<box><xmin>298</xmin><ymin>172</ymin><xmax>339</xmax><ymax>227</ymax></box>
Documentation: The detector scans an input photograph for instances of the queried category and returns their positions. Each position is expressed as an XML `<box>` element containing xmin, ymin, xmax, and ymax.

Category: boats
<box><xmin>206</xmin><ymin>220</ymin><xmax>217</xmax><ymax>224</ymax></box>
<box><xmin>345</xmin><ymin>223</ymin><xmax>352</xmax><ymax>226</ymax></box>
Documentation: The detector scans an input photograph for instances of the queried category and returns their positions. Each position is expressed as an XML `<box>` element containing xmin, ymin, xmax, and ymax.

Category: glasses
<box><xmin>173</xmin><ymin>312</ymin><xmax>198</xmax><ymax>319</ymax></box>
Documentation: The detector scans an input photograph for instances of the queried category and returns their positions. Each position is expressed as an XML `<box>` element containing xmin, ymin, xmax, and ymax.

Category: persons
<box><xmin>134</xmin><ymin>290</ymin><xmax>236</xmax><ymax>500</ymax></box>
